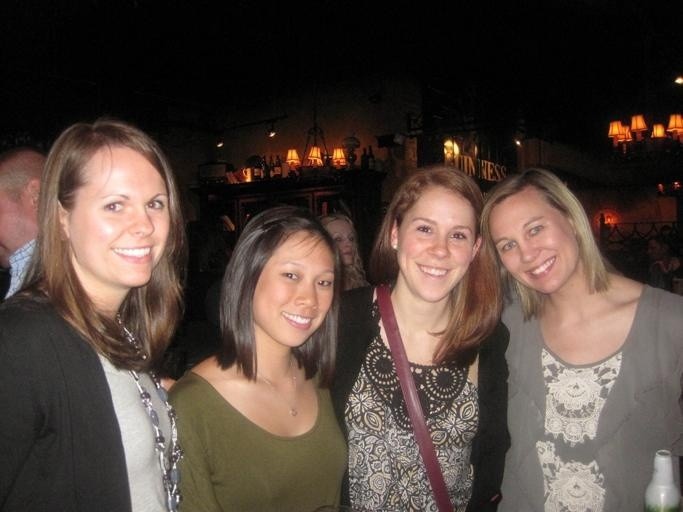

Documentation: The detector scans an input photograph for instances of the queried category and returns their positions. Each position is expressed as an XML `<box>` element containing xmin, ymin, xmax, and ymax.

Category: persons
<box><xmin>313</xmin><ymin>213</ymin><xmax>370</xmax><ymax>296</ymax></box>
<box><xmin>0</xmin><ymin>116</ymin><xmax>195</xmax><ymax>511</ymax></box>
<box><xmin>153</xmin><ymin>200</ymin><xmax>352</xmax><ymax>511</ymax></box>
<box><xmin>645</xmin><ymin>234</ymin><xmax>680</xmax><ymax>294</ymax></box>
<box><xmin>471</xmin><ymin>166</ymin><xmax>682</xmax><ymax>511</ymax></box>
<box><xmin>0</xmin><ymin>145</ymin><xmax>48</xmax><ymax>299</ymax></box>
<box><xmin>282</xmin><ymin>163</ymin><xmax>513</xmax><ymax>511</ymax></box>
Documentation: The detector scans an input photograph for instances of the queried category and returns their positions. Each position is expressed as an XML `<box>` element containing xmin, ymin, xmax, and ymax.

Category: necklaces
<box><xmin>93</xmin><ymin>315</ymin><xmax>186</xmax><ymax>508</ymax></box>
<box><xmin>253</xmin><ymin>359</ymin><xmax>299</xmax><ymax>417</ymax></box>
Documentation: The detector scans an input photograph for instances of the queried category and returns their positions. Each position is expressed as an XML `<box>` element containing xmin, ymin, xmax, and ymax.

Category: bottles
<box><xmin>643</xmin><ymin>449</ymin><xmax>682</xmax><ymax>512</ymax></box>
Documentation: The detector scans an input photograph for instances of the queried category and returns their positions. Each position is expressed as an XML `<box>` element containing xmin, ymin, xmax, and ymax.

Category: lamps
<box><xmin>285</xmin><ymin>75</ymin><xmax>349</xmax><ymax>184</ymax></box>
<box><xmin>603</xmin><ymin>2</ymin><xmax>683</xmax><ymax>154</ymax></box>
